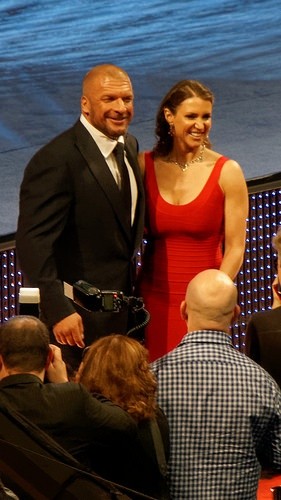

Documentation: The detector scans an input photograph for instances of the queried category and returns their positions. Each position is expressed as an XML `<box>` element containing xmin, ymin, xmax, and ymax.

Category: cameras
<box><xmin>19</xmin><ymin>287</ymin><xmax>40</xmax><ymax>320</ymax></box>
<box><xmin>63</xmin><ymin>280</ymin><xmax>145</xmax><ymax>343</ymax></box>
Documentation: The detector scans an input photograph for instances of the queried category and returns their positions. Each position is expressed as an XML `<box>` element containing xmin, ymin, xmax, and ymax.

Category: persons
<box><xmin>17</xmin><ymin>64</ymin><xmax>146</xmax><ymax>376</ymax></box>
<box><xmin>0</xmin><ymin>225</ymin><xmax>281</xmax><ymax>500</ymax></box>
<box><xmin>138</xmin><ymin>81</ymin><xmax>250</xmax><ymax>364</ymax></box>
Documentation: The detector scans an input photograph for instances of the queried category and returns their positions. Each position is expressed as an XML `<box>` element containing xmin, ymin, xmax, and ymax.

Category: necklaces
<box><xmin>167</xmin><ymin>144</ymin><xmax>205</xmax><ymax>172</ymax></box>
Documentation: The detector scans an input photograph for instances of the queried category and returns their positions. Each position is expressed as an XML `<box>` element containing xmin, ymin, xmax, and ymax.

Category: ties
<box><xmin>113</xmin><ymin>142</ymin><xmax>131</xmax><ymax>227</ymax></box>
